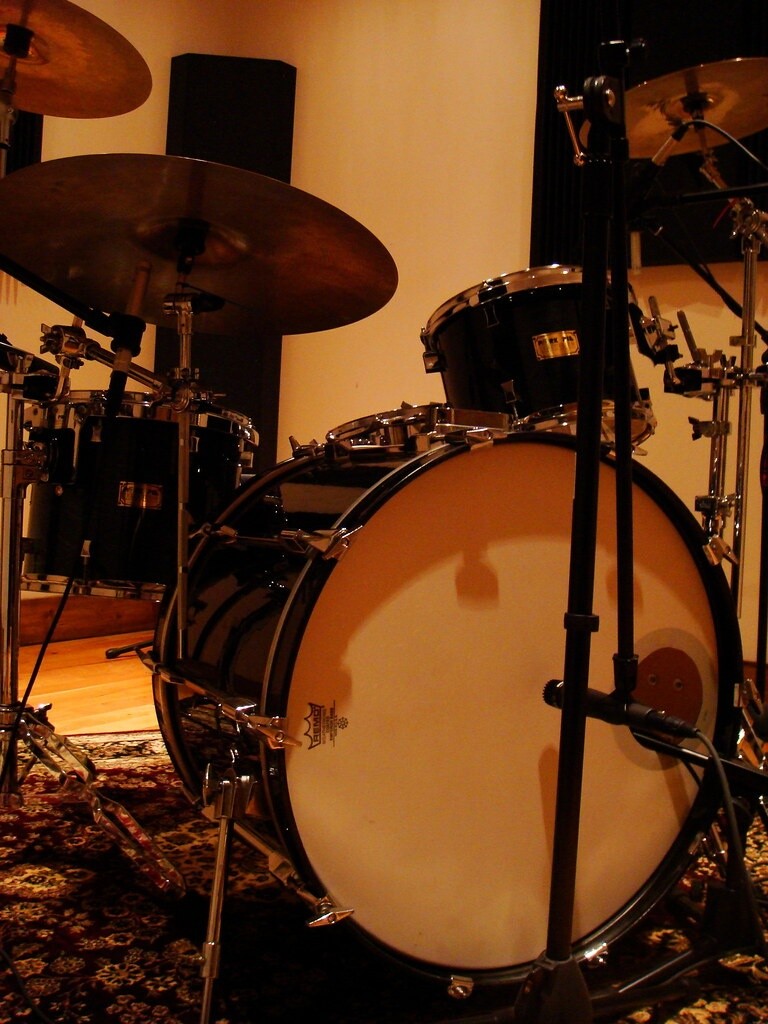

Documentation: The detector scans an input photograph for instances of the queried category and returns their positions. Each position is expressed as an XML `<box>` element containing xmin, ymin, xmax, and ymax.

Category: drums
<box><xmin>425</xmin><ymin>260</ymin><xmax>665</xmax><ymax>449</ymax></box>
<box><xmin>152</xmin><ymin>404</ymin><xmax>747</xmax><ymax>994</ymax></box>
<box><xmin>0</xmin><ymin>342</ymin><xmax>72</xmax><ymax>600</ymax></box>
<box><xmin>21</xmin><ymin>391</ymin><xmax>261</xmax><ymax>602</ymax></box>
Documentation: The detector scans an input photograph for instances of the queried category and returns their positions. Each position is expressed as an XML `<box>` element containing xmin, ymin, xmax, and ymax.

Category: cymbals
<box><xmin>578</xmin><ymin>56</ymin><xmax>768</xmax><ymax>159</ymax></box>
<box><xmin>0</xmin><ymin>0</ymin><xmax>153</xmax><ymax>119</ymax></box>
<box><xmin>0</xmin><ymin>152</ymin><xmax>399</xmax><ymax>337</ymax></box>
